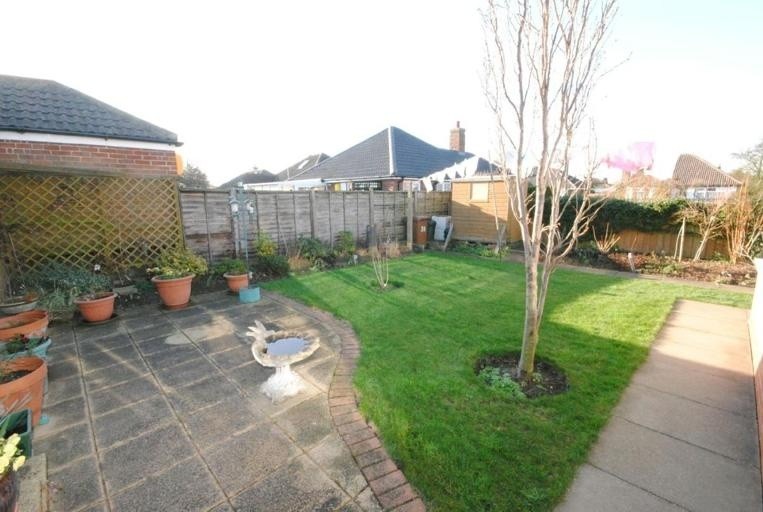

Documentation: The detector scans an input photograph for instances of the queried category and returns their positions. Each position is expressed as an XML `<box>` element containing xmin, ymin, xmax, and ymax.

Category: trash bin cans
<box><xmin>413</xmin><ymin>215</ymin><xmax>436</xmax><ymax>252</ymax></box>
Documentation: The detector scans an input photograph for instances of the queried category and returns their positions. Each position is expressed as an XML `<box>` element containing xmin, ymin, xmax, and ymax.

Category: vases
<box><xmin>0</xmin><ymin>311</ymin><xmax>48</xmax><ymax>511</ymax></box>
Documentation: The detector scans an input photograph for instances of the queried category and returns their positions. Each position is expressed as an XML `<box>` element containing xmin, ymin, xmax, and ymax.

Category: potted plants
<box><xmin>149</xmin><ymin>247</ymin><xmax>208</xmax><ymax>308</ymax></box>
<box><xmin>218</xmin><ymin>255</ymin><xmax>252</xmax><ymax>293</ymax></box>
<box><xmin>1</xmin><ymin>268</ymin><xmax>135</xmax><ymax>326</ymax></box>
